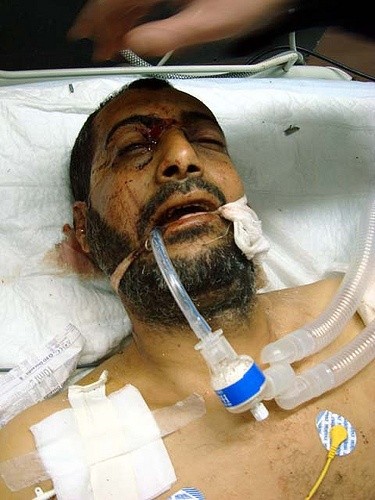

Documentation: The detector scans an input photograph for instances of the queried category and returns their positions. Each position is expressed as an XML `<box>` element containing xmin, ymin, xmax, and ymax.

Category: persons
<box><xmin>66</xmin><ymin>0</ymin><xmax>297</xmax><ymax>63</ymax></box>
<box><xmin>0</xmin><ymin>78</ymin><xmax>375</xmax><ymax>500</ymax></box>
